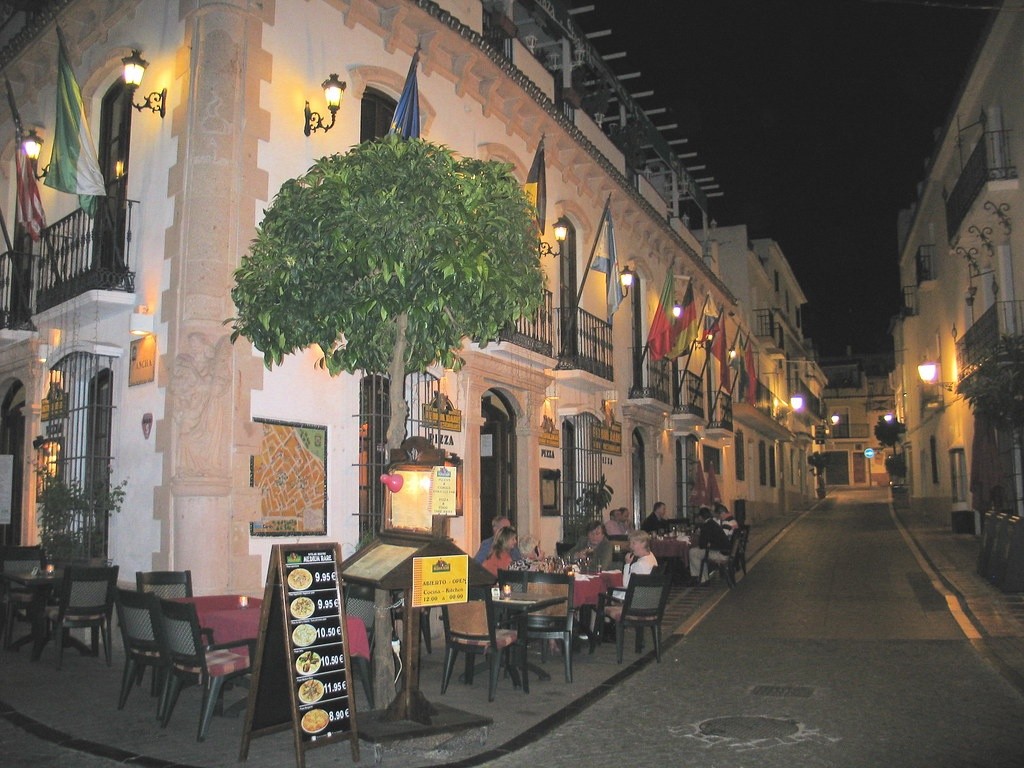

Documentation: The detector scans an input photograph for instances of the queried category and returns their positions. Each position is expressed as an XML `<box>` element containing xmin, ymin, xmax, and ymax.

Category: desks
<box><xmin>569</xmin><ymin>570</ymin><xmax>622</xmax><ymax>628</ymax></box>
<box><xmin>177</xmin><ymin>595</ymin><xmax>376</xmax><ymax>715</ymax></box>
<box><xmin>649</xmin><ymin>533</ymin><xmax>694</xmax><ymax>569</ymax></box>
<box><xmin>3</xmin><ymin>569</ymin><xmax>92</xmax><ymax>665</ymax></box>
<box><xmin>460</xmin><ymin>593</ymin><xmax>568</xmax><ymax>685</ymax></box>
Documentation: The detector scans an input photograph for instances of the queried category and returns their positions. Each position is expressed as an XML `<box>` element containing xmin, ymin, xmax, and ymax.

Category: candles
<box><xmin>46</xmin><ymin>564</ymin><xmax>54</xmax><ymax>576</ymax></box>
<box><xmin>239</xmin><ymin>597</ymin><xmax>249</xmax><ymax>608</ymax></box>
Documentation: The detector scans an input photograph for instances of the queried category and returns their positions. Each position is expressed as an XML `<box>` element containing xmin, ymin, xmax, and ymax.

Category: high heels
<box><xmin>549</xmin><ymin>639</ymin><xmax>562</xmax><ymax>656</ymax></box>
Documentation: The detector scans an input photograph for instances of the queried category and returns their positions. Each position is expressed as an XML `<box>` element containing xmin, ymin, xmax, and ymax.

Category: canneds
<box><xmin>596</xmin><ymin>565</ymin><xmax>602</xmax><ymax>574</ymax></box>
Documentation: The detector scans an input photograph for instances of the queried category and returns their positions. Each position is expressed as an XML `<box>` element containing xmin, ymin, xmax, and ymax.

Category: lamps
<box><xmin>33</xmin><ymin>436</ymin><xmax>45</xmax><ymax>450</ymax></box>
<box><xmin>621</xmin><ymin>264</ymin><xmax>635</xmax><ymax>298</ymax></box>
<box><xmin>917</xmin><ymin>354</ymin><xmax>953</xmax><ymax>392</ymax></box>
<box><xmin>128</xmin><ymin>313</ymin><xmax>154</xmax><ymax>336</ymax></box>
<box><xmin>39</xmin><ymin>344</ymin><xmax>49</xmax><ymax>362</ymax></box>
<box><xmin>23</xmin><ymin>129</ymin><xmax>52</xmax><ymax>179</ymax></box>
<box><xmin>536</xmin><ymin>215</ymin><xmax>568</xmax><ymax>258</ymax></box>
<box><xmin>304</xmin><ymin>73</ymin><xmax>347</xmax><ymax>137</ymax></box>
<box><xmin>121</xmin><ymin>47</ymin><xmax>167</xmax><ymax>118</ymax></box>
<box><xmin>380</xmin><ymin>457</ymin><xmax>419</xmax><ymax>493</ymax></box>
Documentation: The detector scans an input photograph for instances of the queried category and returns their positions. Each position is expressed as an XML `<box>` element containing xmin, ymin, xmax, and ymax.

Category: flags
<box><xmin>41</xmin><ymin>41</ymin><xmax>107</xmax><ymax>219</ymax></box>
<box><xmin>15</xmin><ymin>118</ymin><xmax>47</xmax><ymax>241</ymax></box>
<box><xmin>696</xmin><ymin>297</ymin><xmax>756</xmax><ymax>406</ymax></box>
<box><xmin>390</xmin><ymin>57</ymin><xmax>419</xmax><ymax>139</ymax></box>
<box><xmin>648</xmin><ymin>268</ymin><xmax>699</xmax><ymax>363</ymax></box>
<box><xmin>590</xmin><ymin>208</ymin><xmax>623</xmax><ymax>326</ymax></box>
<box><xmin>523</xmin><ymin>147</ymin><xmax>547</xmax><ymax>237</ymax></box>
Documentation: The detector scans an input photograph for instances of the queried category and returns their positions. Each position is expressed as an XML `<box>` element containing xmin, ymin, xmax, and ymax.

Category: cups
<box><xmin>545</xmin><ymin>544</ymin><xmax>594</xmax><ymax>578</ymax></box>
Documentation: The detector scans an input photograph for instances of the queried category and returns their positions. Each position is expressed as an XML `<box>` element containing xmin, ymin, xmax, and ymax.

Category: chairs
<box><xmin>0</xmin><ymin>545</ymin><xmax>259</xmax><ymax>741</ymax></box>
<box><xmin>439</xmin><ymin>519</ymin><xmax>750</xmax><ymax>703</ymax></box>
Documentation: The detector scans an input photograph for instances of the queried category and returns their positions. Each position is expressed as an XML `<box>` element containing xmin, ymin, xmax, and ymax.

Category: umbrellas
<box><xmin>690</xmin><ymin>460</ymin><xmax>722</xmax><ymax>509</ymax></box>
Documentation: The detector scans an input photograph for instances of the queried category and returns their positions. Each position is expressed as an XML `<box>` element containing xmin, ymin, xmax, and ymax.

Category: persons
<box><xmin>641</xmin><ymin>502</ymin><xmax>668</xmax><ymax>532</ymax></box>
<box><xmin>603</xmin><ymin>507</ymin><xmax>634</xmax><ymax>536</ymax></box>
<box><xmin>685</xmin><ymin>504</ymin><xmax>738</xmax><ymax>586</ymax></box>
<box><xmin>564</xmin><ymin>520</ymin><xmax>613</xmax><ymax>573</ymax></box>
<box><xmin>578</xmin><ymin>530</ymin><xmax>658</xmax><ymax>640</ymax></box>
<box><xmin>472</xmin><ymin>515</ymin><xmax>544</xmax><ymax>578</ymax></box>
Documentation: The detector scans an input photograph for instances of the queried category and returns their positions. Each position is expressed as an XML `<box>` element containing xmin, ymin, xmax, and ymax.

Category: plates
<box><xmin>288</xmin><ymin>567</ymin><xmax>333</xmax><ymax>735</ymax></box>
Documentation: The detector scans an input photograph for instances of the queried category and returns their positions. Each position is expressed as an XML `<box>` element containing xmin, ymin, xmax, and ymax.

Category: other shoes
<box><xmin>700</xmin><ymin>581</ymin><xmax>707</xmax><ymax>587</ymax></box>
<box><xmin>579</xmin><ymin>634</ymin><xmax>589</xmax><ymax>640</ymax></box>
<box><xmin>706</xmin><ymin>580</ymin><xmax>710</xmax><ymax>585</ymax></box>
